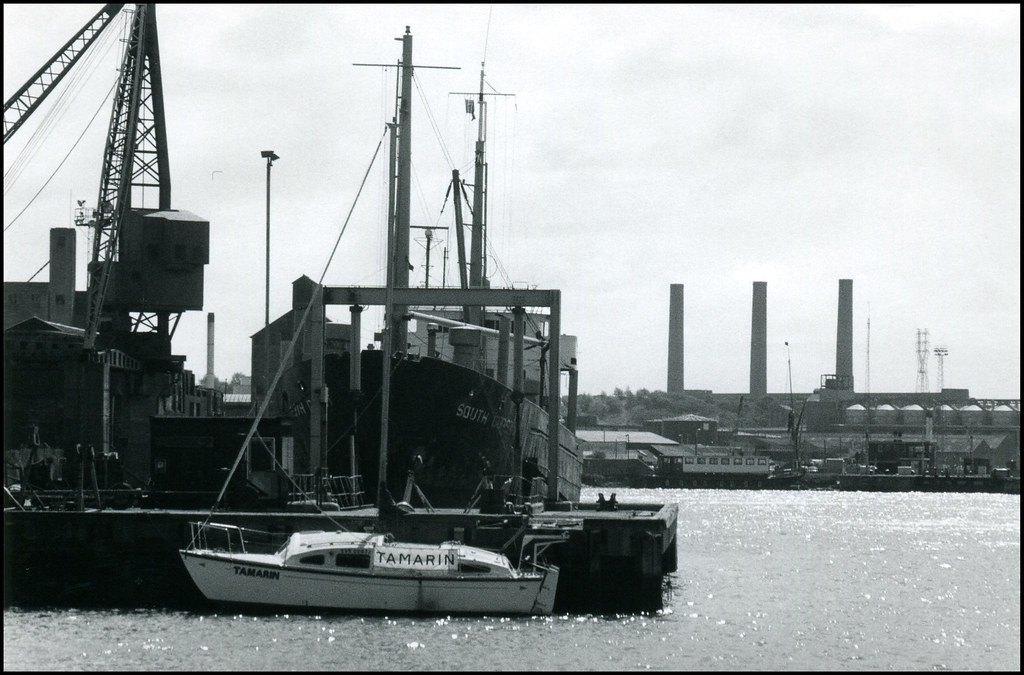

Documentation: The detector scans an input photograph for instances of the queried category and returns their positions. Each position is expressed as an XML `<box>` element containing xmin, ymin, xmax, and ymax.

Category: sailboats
<box><xmin>176</xmin><ymin>23</ymin><xmax>549</xmax><ymax>619</ymax></box>
<box><xmin>765</xmin><ymin>320</ymin><xmax>843</xmax><ymax>491</ymax></box>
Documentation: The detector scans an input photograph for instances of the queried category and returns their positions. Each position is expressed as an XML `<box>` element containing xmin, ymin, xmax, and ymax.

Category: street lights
<box><xmin>261</xmin><ymin>149</ymin><xmax>280</xmax><ymax>417</ymax></box>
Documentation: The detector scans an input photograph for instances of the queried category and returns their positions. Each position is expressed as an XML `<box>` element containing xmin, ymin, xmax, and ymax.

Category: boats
<box><xmin>291</xmin><ymin>62</ymin><xmax>585</xmax><ymax>502</ymax></box>
